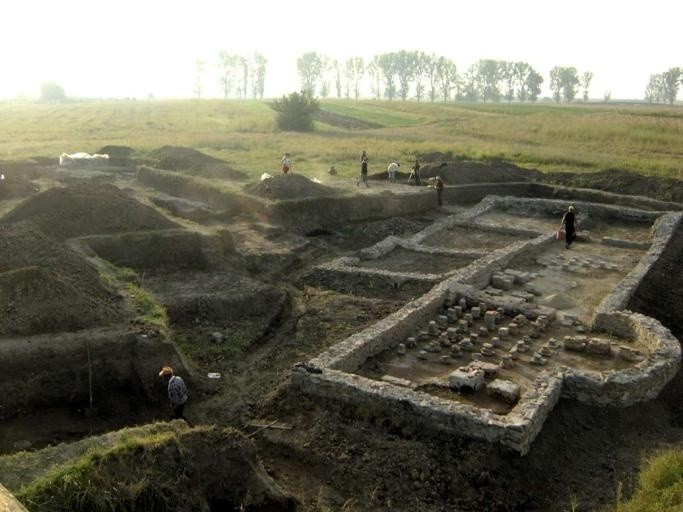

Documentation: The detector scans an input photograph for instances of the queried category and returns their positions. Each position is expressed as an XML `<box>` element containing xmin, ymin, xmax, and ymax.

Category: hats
<box><xmin>158</xmin><ymin>366</ymin><xmax>173</xmax><ymax>376</ymax></box>
<box><xmin>569</xmin><ymin>206</ymin><xmax>575</xmax><ymax>211</ymax></box>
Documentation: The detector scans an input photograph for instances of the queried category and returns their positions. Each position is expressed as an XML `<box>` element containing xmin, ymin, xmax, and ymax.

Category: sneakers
<box><xmin>565</xmin><ymin>242</ymin><xmax>569</xmax><ymax>249</ymax></box>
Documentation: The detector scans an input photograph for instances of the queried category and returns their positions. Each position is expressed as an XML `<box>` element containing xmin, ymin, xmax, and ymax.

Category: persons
<box><xmin>436</xmin><ymin>176</ymin><xmax>443</xmax><ymax>206</ymax></box>
<box><xmin>355</xmin><ymin>149</ymin><xmax>372</xmax><ymax>191</ymax></box>
<box><xmin>279</xmin><ymin>152</ymin><xmax>292</xmax><ymax>174</ymax></box>
<box><xmin>388</xmin><ymin>162</ymin><xmax>400</xmax><ymax>183</ymax></box>
<box><xmin>559</xmin><ymin>205</ymin><xmax>583</xmax><ymax>250</ymax></box>
<box><xmin>158</xmin><ymin>366</ymin><xmax>189</xmax><ymax>420</ymax></box>
<box><xmin>412</xmin><ymin>159</ymin><xmax>422</xmax><ymax>185</ymax></box>
